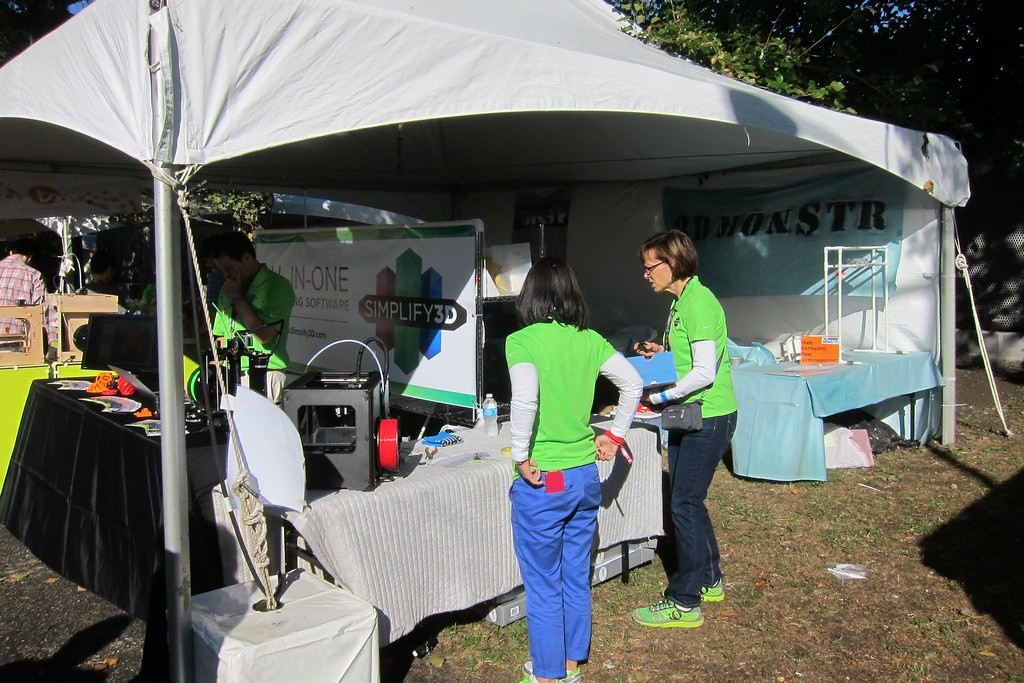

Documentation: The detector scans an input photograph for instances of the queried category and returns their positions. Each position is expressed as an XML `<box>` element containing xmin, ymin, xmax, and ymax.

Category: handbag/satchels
<box><xmin>661</xmin><ymin>400</ymin><xmax>703</xmax><ymax>430</ymax></box>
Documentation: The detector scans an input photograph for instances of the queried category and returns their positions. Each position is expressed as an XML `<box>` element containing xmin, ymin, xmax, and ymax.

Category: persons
<box><xmin>633</xmin><ymin>229</ymin><xmax>738</xmax><ymax>628</ymax></box>
<box><xmin>505</xmin><ymin>258</ymin><xmax>644</xmax><ymax>683</ymax></box>
<box><xmin>202</xmin><ymin>229</ymin><xmax>295</xmax><ymax>409</ymax></box>
<box><xmin>1</xmin><ymin>232</ymin><xmax>158</xmax><ymax>316</ymax></box>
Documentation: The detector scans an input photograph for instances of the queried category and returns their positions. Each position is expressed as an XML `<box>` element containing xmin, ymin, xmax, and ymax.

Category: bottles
<box><xmin>482</xmin><ymin>393</ymin><xmax>499</xmax><ymax>441</ymax></box>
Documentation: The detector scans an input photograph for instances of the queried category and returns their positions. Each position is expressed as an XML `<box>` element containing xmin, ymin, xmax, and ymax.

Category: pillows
<box><xmin>608</xmin><ymin>333</ymin><xmax>656</xmax><ymax>355</ymax></box>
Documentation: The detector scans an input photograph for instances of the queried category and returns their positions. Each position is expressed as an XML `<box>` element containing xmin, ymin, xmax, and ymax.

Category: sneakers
<box><xmin>659</xmin><ymin>578</ymin><xmax>724</xmax><ymax>601</ymax></box>
<box><xmin>633</xmin><ymin>598</ymin><xmax>704</xmax><ymax>628</ymax></box>
<box><xmin>517</xmin><ymin>661</ymin><xmax>582</xmax><ymax>683</ymax></box>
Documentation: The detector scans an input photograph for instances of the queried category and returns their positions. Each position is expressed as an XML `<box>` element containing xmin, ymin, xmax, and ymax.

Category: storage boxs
<box><xmin>474</xmin><ymin>537</ymin><xmax>657</xmax><ymax>627</ymax></box>
<box><xmin>0</xmin><ymin>293</ymin><xmax>119</xmax><ymax>366</ymax></box>
<box><xmin>627</xmin><ymin>351</ymin><xmax>677</xmax><ymax>390</ymax></box>
<box><xmin>610</xmin><ymin>411</ymin><xmax>669</xmax><ymax>444</ymax></box>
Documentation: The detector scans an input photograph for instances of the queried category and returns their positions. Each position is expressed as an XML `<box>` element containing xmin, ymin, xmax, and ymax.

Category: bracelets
<box><xmin>659</xmin><ymin>386</ymin><xmax>669</xmax><ymax>404</ymax></box>
<box><xmin>229</xmin><ymin>291</ymin><xmax>241</xmax><ymax>304</ymax></box>
<box><xmin>605</xmin><ymin>431</ymin><xmax>624</xmax><ymax>446</ymax></box>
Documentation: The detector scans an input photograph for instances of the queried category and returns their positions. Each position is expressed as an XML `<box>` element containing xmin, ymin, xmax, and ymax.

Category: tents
<box><xmin>0</xmin><ymin>2</ymin><xmax>1010</xmax><ymax>683</ymax></box>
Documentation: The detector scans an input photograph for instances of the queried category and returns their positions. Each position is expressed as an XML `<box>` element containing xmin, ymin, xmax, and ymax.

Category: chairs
<box><xmin>614</xmin><ymin>326</ymin><xmax>658</xmax><ymax>357</ymax></box>
<box><xmin>785</xmin><ymin>336</ymin><xmax>802</xmax><ymax>362</ymax></box>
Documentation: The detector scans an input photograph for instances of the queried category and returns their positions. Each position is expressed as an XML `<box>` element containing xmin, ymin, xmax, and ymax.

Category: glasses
<box><xmin>644</xmin><ymin>260</ymin><xmax>665</xmax><ymax>275</ymax></box>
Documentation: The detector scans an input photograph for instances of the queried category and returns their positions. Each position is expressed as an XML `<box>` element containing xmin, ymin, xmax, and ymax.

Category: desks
<box><xmin>211</xmin><ymin>412</ymin><xmax>665</xmax><ymax>647</ymax></box>
<box><xmin>0</xmin><ymin>376</ymin><xmax>286</xmax><ymax>622</ymax></box>
<box><xmin>730</xmin><ymin>350</ymin><xmax>946</xmax><ymax>481</ymax></box>
<box><xmin>167</xmin><ymin>569</ymin><xmax>381</xmax><ymax>683</ymax></box>
<box><xmin>0</xmin><ymin>343</ymin><xmax>209</xmax><ymax>496</ymax></box>
